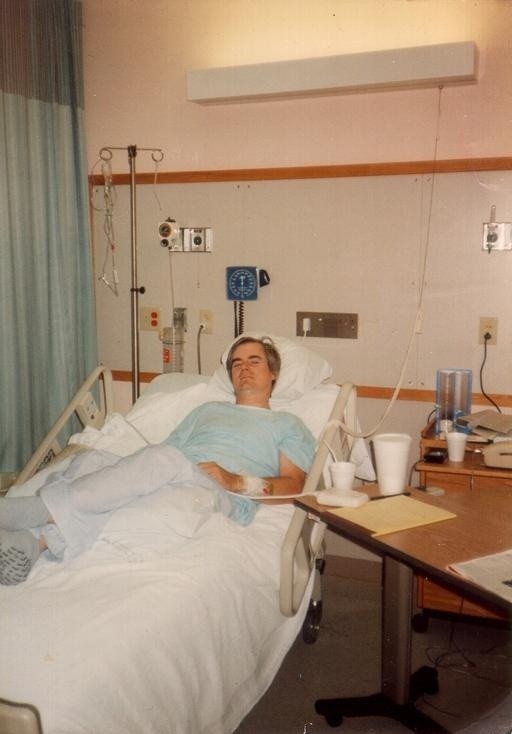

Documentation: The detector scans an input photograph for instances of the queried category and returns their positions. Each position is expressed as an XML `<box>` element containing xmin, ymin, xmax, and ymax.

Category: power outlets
<box><xmin>199</xmin><ymin>308</ymin><xmax>214</xmax><ymax>335</ymax></box>
<box><xmin>295</xmin><ymin>310</ymin><xmax>358</xmax><ymax>340</ymax></box>
<box><xmin>479</xmin><ymin>315</ymin><xmax>497</xmax><ymax>344</ymax></box>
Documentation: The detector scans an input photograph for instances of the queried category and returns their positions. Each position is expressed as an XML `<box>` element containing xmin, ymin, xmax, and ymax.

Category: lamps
<box><xmin>184</xmin><ymin>40</ymin><xmax>481</xmax><ymax>106</ymax></box>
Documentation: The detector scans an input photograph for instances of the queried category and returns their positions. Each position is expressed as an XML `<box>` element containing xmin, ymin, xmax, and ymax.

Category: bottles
<box><xmin>160</xmin><ymin>325</ymin><xmax>185</xmax><ymax>371</ymax></box>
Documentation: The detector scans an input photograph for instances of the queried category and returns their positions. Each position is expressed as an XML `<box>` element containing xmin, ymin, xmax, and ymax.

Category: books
<box><xmin>326</xmin><ymin>494</ymin><xmax>457</xmax><ymax>538</ymax></box>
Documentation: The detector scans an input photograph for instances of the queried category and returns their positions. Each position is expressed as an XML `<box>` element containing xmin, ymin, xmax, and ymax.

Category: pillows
<box><xmin>211</xmin><ymin>330</ymin><xmax>335</xmax><ymax>404</ymax></box>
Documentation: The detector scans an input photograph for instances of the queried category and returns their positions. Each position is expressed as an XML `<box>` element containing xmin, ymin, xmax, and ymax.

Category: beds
<box><xmin>0</xmin><ymin>365</ymin><xmax>363</xmax><ymax>734</ymax></box>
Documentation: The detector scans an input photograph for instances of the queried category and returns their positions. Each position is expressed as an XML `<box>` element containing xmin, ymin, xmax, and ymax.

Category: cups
<box><xmin>433</xmin><ymin>366</ymin><xmax>471</xmax><ymax>438</ymax></box>
<box><xmin>373</xmin><ymin>432</ymin><xmax>411</xmax><ymax>495</ymax></box>
<box><xmin>330</xmin><ymin>462</ymin><xmax>355</xmax><ymax>488</ymax></box>
<box><xmin>446</xmin><ymin>431</ymin><xmax>467</xmax><ymax>463</ymax></box>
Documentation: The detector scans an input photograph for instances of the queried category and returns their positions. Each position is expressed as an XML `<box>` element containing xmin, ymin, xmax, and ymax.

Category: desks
<box><xmin>294</xmin><ymin>489</ymin><xmax>512</xmax><ymax>734</ymax></box>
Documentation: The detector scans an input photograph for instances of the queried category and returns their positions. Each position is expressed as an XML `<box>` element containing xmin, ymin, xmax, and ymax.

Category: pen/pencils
<box><xmin>370</xmin><ymin>493</ymin><xmax>411</xmax><ymax>500</ymax></box>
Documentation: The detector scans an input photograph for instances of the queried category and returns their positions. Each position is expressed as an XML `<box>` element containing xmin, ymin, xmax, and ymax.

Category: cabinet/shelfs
<box><xmin>413</xmin><ymin>421</ymin><xmax>512</xmax><ymax>620</ymax></box>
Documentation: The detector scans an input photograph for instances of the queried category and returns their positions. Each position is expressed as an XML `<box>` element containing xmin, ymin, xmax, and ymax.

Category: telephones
<box><xmin>482</xmin><ymin>441</ymin><xmax>512</xmax><ymax>468</ymax></box>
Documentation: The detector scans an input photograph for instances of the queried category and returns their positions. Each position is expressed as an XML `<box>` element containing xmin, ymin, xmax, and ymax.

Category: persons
<box><xmin>164</xmin><ymin>337</ymin><xmax>317</xmax><ymax>505</ymax></box>
